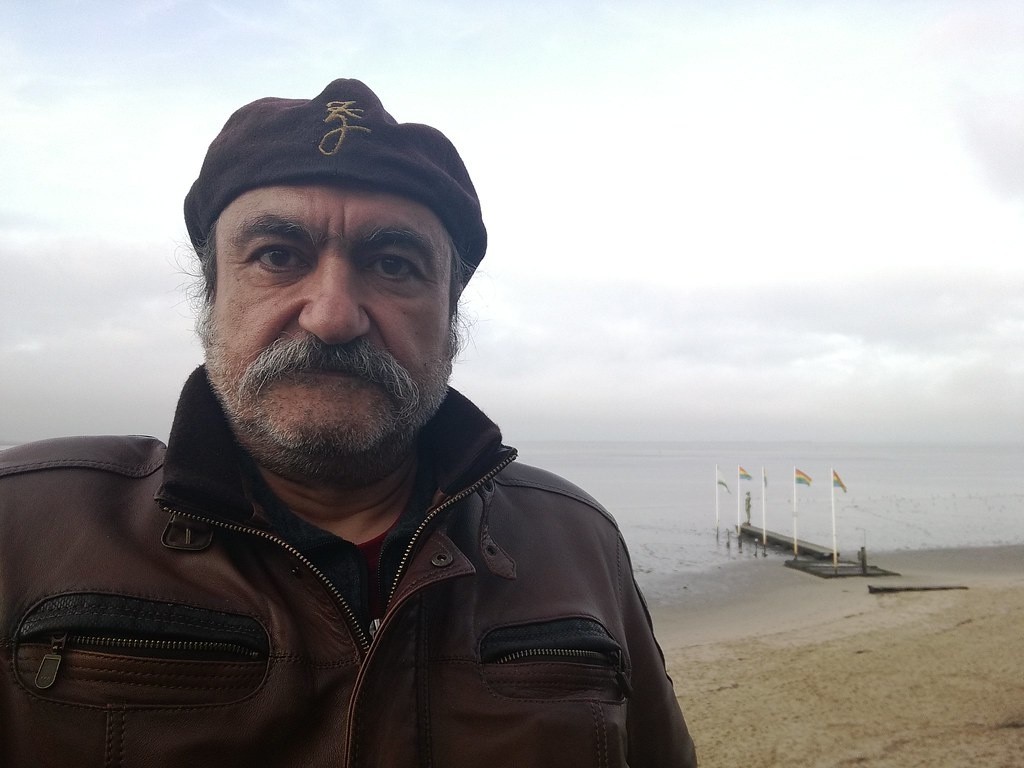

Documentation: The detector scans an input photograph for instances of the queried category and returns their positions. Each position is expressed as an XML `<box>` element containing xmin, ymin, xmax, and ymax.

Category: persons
<box><xmin>0</xmin><ymin>78</ymin><xmax>696</xmax><ymax>768</ymax></box>
<box><xmin>745</xmin><ymin>492</ymin><xmax>751</xmax><ymax>524</ymax></box>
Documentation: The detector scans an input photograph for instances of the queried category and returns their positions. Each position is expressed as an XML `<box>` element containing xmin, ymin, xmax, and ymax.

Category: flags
<box><xmin>795</xmin><ymin>468</ymin><xmax>812</xmax><ymax>486</ymax></box>
<box><xmin>717</xmin><ymin>465</ymin><xmax>730</xmax><ymax>494</ymax></box>
<box><xmin>833</xmin><ymin>470</ymin><xmax>847</xmax><ymax>493</ymax></box>
<box><xmin>739</xmin><ymin>466</ymin><xmax>752</xmax><ymax>480</ymax></box>
<box><xmin>764</xmin><ymin>468</ymin><xmax>768</xmax><ymax>487</ymax></box>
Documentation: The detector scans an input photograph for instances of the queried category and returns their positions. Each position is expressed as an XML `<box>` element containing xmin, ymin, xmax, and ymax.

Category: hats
<box><xmin>184</xmin><ymin>78</ymin><xmax>488</xmax><ymax>301</ymax></box>
